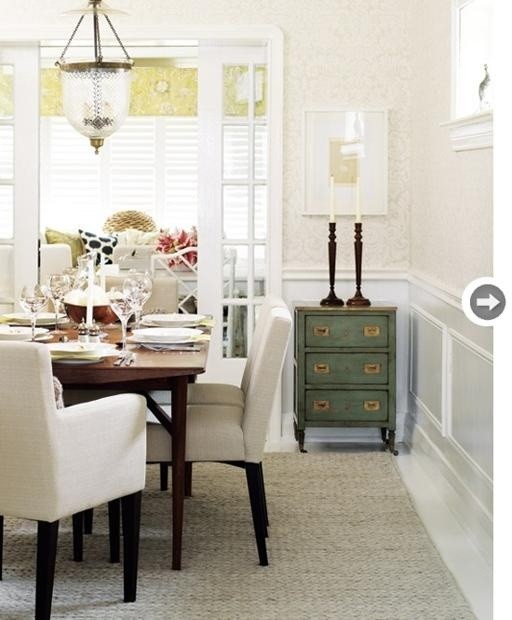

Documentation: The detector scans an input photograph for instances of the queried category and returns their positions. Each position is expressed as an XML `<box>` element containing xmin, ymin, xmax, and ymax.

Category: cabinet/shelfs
<box><xmin>292</xmin><ymin>305</ymin><xmax>403</xmax><ymax>458</ymax></box>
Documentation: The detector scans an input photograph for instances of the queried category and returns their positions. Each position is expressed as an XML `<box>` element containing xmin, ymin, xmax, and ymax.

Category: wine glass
<box><xmin>20</xmin><ymin>286</ymin><xmax>47</xmax><ymax>342</ymax></box>
<box><xmin>48</xmin><ymin>273</ymin><xmax>68</xmax><ymax>335</ymax></box>
<box><xmin>110</xmin><ymin>284</ymin><xmax>135</xmax><ymax>356</ymax></box>
<box><xmin>123</xmin><ymin>273</ymin><xmax>152</xmax><ymax>340</ymax></box>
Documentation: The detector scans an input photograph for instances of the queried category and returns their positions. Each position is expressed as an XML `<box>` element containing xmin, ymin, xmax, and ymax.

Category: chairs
<box><xmin>38</xmin><ymin>241</ymin><xmax>74</xmax><ymax>318</ymax></box>
<box><xmin>138</xmin><ymin>303</ymin><xmax>295</xmax><ymax>571</ymax></box>
<box><xmin>152</xmin><ymin>248</ymin><xmax>235</xmax><ymax>359</ymax></box>
<box><xmin>2</xmin><ymin>338</ymin><xmax>149</xmax><ymax>617</ymax></box>
<box><xmin>106</xmin><ymin>277</ymin><xmax>185</xmax><ymax>324</ymax></box>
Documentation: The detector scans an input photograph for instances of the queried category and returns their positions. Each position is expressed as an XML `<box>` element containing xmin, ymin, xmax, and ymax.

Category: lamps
<box><xmin>53</xmin><ymin>0</ymin><xmax>131</xmax><ymax>155</ymax></box>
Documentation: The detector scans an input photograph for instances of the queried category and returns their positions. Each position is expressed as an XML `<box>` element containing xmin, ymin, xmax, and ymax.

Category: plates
<box><xmin>139</xmin><ymin>321</ymin><xmax>213</xmax><ymax>330</ymax></box>
<box><xmin>7</xmin><ymin>321</ymin><xmax>73</xmax><ymax>327</ymax></box>
<box><xmin>144</xmin><ymin>313</ymin><xmax>205</xmax><ymax>326</ymax></box>
<box><xmin>10</xmin><ymin>311</ymin><xmax>67</xmax><ymax>324</ymax></box>
<box><xmin>49</xmin><ymin>351</ymin><xmax>122</xmax><ymax>359</ymax></box>
<box><xmin>129</xmin><ymin>327</ymin><xmax>203</xmax><ymax>339</ymax></box>
<box><xmin>0</xmin><ymin>335</ymin><xmax>55</xmax><ymax>344</ymax></box>
<box><xmin>48</xmin><ymin>341</ymin><xmax>118</xmax><ymax>357</ymax></box>
<box><xmin>0</xmin><ymin>326</ymin><xmax>49</xmax><ymax>341</ymax></box>
<box><xmin>125</xmin><ymin>336</ymin><xmax>208</xmax><ymax>351</ymax></box>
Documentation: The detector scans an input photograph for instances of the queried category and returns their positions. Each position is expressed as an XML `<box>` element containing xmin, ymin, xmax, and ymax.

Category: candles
<box><xmin>351</xmin><ymin>184</ymin><xmax>374</xmax><ymax>312</ymax></box>
<box><xmin>321</xmin><ymin>173</ymin><xmax>343</xmax><ymax>308</ymax></box>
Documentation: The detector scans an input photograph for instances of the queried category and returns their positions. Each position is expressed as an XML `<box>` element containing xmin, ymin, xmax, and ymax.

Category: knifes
<box><xmin>115</xmin><ymin>349</ymin><xmax>124</xmax><ymax>366</ymax></box>
<box><xmin>125</xmin><ymin>351</ymin><xmax>135</xmax><ymax>366</ymax></box>
